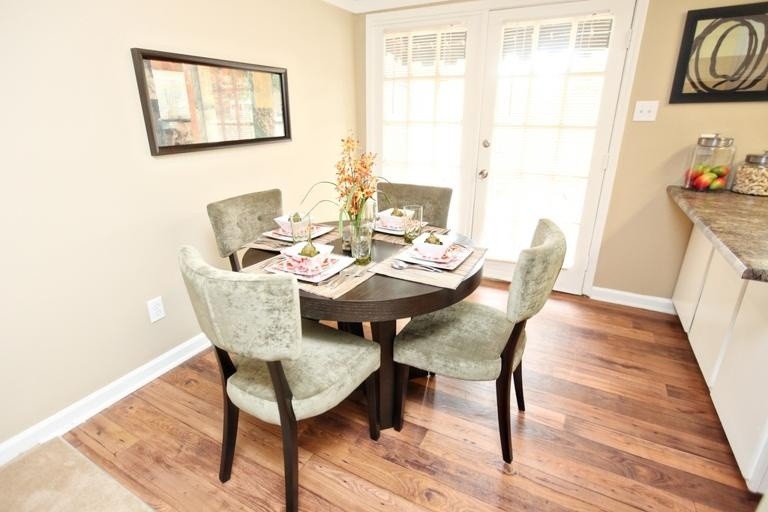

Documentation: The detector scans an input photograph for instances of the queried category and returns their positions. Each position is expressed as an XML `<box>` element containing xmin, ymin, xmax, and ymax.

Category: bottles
<box><xmin>683</xmin><ymin>134</ymin><xmax>737</xmax><ymax>191</ymax></box>
<box><xmin>730</xmin><ymin>151</ymin><xmax>768</xmax><ymax>197</ymax></box>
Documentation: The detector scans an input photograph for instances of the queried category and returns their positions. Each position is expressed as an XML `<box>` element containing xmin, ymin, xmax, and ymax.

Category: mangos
<box><xmin>685</xmin><ymin>162</ymin><xmax>731</xmax><ymax>192</ymax></box>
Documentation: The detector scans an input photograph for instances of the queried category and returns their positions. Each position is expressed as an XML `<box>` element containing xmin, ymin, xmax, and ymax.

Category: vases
<box><xmin>345</xmin><ymin>216</ymin><xmax>366</xmax><ymax>244</ymax></box>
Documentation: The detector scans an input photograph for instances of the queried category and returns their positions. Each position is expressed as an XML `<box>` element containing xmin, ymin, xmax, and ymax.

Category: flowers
<box><xmin>301</xmin><ymin>132</ymin><xmax>389</xmax><ymax>240</ymax></box>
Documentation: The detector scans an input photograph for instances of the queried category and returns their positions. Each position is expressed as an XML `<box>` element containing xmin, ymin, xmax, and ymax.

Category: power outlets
<box><xmin>633</xmin><ymin>100</ymin><xmax>659</xmax><ymax>122</ymax></box>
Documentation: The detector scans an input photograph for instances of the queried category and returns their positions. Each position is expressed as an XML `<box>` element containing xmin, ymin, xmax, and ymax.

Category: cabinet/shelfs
<box><xmin>668</xmin><ymin>224</ymin><xmax>768</xmax><ymax>496</ymax></box>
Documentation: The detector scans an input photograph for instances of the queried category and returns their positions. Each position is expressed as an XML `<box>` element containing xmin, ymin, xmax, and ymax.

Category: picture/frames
<box><xmin>669</xmin><ymin>4</ymin><xmax>767</xmax><ymax>102</ymax></box>
<box><xmin>132</xmin><ymin>47</ymin><xmax>291</xmax><ymax>155</ymax></box>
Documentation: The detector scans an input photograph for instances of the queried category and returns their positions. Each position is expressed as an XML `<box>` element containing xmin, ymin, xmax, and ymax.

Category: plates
<box><xmin>363</xmin><ymin>220</ymin><xmax>429</xmax><ymax>236</ymax></box>
<box><xmin>395</xmin><ymin>232</ymin><xmax>475</xmax><ymax>271</ymax></box>
<box><xmin>263</xmin><ymin>253</ymin><xmax>357</xmax><ymax>284</ymax></box>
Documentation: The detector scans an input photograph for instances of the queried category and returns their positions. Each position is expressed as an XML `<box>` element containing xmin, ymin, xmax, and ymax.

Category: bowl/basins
<box><xmin>273</xmin><ymin>212</ymin><xmax>320</xmax><ymax>232</ymax></box>
<box><xmin>377</xmin><ymin>208</ymin><xmax>415</xmax><ymax>228</ymax></box>
<box><xmin>412</xmin><ymin>232</ymin><xmax>456</xmax><ymax>258</ymax></box>
<box><xmin>279</xmin><ymin>241</ymin><xmax>335</xmax><ymax>270</ymax></box>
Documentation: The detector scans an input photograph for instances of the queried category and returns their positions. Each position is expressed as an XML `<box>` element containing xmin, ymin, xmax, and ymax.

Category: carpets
<box><xmin>0</xmin><ymin>437</ymin><xmax>157</xmax><ymax>512</ymax></box>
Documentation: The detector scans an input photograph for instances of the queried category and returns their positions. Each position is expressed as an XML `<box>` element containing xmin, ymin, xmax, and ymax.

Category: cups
<box><xmin>348</xmin><ymin>221</ymin><xmax>372</xmax><ymax>265</ymax></box>
<box><xmin>403</xmin><ymin>204</ymin><xmax>424</xmax><ymax>243</ymax></box>
<box><xmin>290</xmin><ymin>215</ymin><xmax>312</xmax><ymax>244</ymax></box>
<box><xmin>362</xmin><ymin>201</ymin><xmax>377</xmax><ymax>237</ymax></box>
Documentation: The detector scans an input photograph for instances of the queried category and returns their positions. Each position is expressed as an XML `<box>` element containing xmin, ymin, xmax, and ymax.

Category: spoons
<box><xmin>390</xmin><ymin>260</ymin><xmax>434</xmax><ymax>273</ymax></box>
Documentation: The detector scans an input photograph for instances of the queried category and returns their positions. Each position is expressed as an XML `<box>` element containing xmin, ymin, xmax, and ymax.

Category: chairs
<box><xmin>207</xmin><ymin>188</ymin><xmax>282</xmax><ymax>273</ymax></box>
<box><xmin>392</xmin><ymin>217</ymin><xmax>567</xmax><ymax>463</ymax></box>
<box><xmin>177</xmin><ymin>244</ymin><xmax>381</xmax><ymax>511</ymax></box>
<box><xmin>375</xmin><ymin>184</ymin><xmax>452</xmax><ymax>231</ymax></box>
